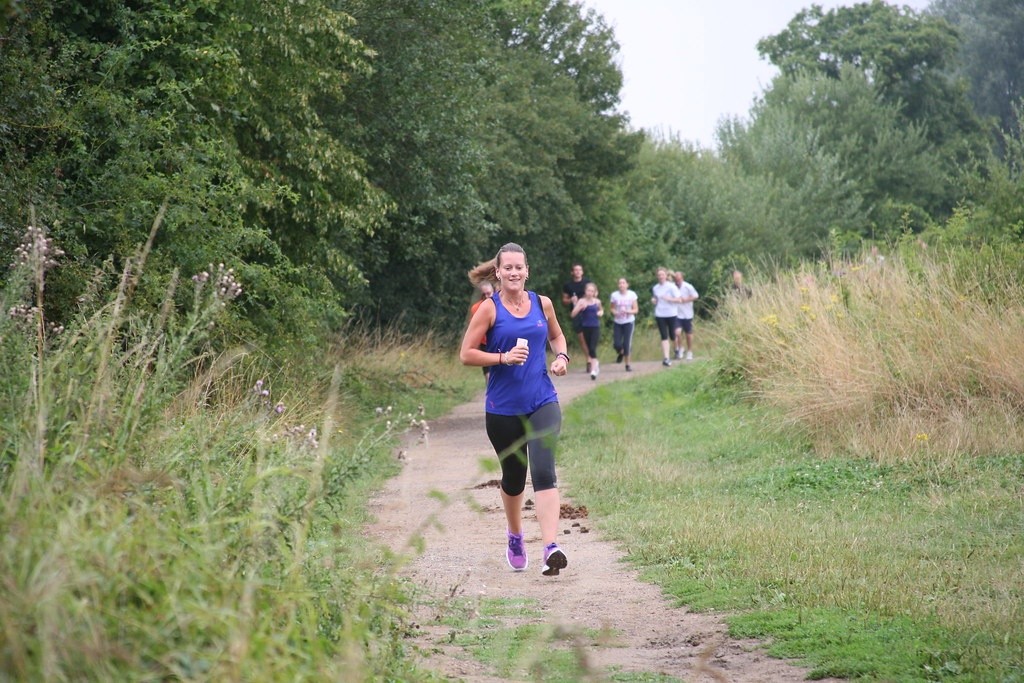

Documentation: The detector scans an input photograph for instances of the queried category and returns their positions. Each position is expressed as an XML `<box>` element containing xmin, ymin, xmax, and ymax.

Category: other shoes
<box><xmin>674</xmin><ymin>348</ymin><xmax>679</xmax><ymax>359</ymax></box>
<box><xmin>678</xmin><ymin>347</ymin><xmax>685</xmax><ymax>358</ymax></box>
<box><xmin>591</xmin><ymin>371</ymin><xmax>596</xmax><ymax>379</ymax></box>
<box><xmin>617</xmin><ymin>354</ymin><xmax>623</xmax><ymax>362</ymax></box>
<box><xmin>626</xmin><ymin>365</ymin><xmax>632</xmax><ymax>371</ymax></box>
<box><xmin>686</xmin><ymin>351</ymin><xmax>693</xmax><ymax>359</ymax></box>
<box><xmin>663</xmin><ymin>358</ymin><xmax>671</xmax><ymax>365</ymax></box>
<box><xmin>587</xmin><ymin>362</ymin><xmax>591</xmax><ymax>372</ymax></box>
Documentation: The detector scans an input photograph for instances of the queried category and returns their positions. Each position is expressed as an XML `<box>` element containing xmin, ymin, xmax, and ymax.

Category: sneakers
<box><xmin>506</xmin><ymin>526</ymin><xmax>528</xmax><ymax>571</ymax></box>
<box><xmin>542</xmin><ymin>542</ymin><xmax>567</xmax><ymax>575</ymax></box>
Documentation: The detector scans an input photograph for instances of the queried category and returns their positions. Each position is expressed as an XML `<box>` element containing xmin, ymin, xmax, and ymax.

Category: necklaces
<box><xmin>502</xmin><ymin>291</ymin><xmax>523</xmax><ymax>311</ymax></box>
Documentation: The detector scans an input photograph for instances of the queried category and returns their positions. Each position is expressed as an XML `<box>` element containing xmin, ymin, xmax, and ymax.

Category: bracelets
<box><xmin>505</xmin><ymin>351</ymin><xmax>513</xmax><ymax>366</ymax></box>
<box><xmin>500</xmin><ymin>353</ymin><xmax>501</xmax><ymax>366</ymax></box>
<box><xmin>556</xmin><ymin>352</ymin><xmax>569</xmax><ymax>364</ymax></box>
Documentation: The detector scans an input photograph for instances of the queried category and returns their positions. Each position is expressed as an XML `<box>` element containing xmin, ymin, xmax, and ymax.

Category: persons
<box><xmin>673</xmin><ymin>271</ymin><xmax>699</xmax><ymax>361</ymax></box>
<box><xmin>731</xmin><ymin>271</ymin><xmax>752</xmax><ymax>302</ymax></box>
<box><xmin>609</xmin><ymin>278</ymin><xmax>638</xmax><ymax>372</ymax></box>
<box><xmin>562</xmin><ymin>264</ymin><xmax>599</xmax><ymax>373</ymax></box>
<box><xmin>570</xmin><ymin>282</ymin><xmax>604</xmax><ymax>380</ymax></box>
<box><xmin>651</xmin><ymin>267</ymin><xmax>683</xmax><ymax>366</ymax></box>
<box><xmin>459</xmin><ymin>242</ymin><xmax>569</xmax><ymax>576</ymax></box>
<box><xmin>470</xmin><ymin>281</ymin><xmax>500</xmax><ymax>389</ymax></box>
